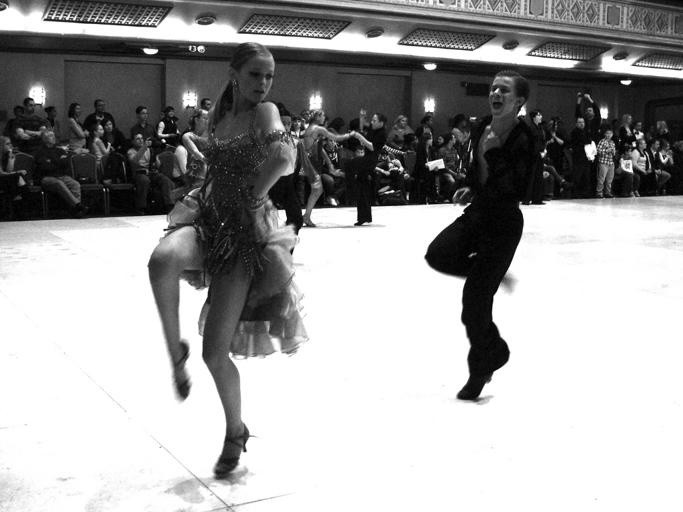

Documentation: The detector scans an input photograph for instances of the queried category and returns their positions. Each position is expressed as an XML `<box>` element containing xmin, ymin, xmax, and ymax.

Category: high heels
<box><xmin>173</xmin><ymin>342</ymin><xmax>191</xmax><ymax>398</ymax></box>
<box><xmin>214</xmin><ymin>424</ymin><xmax>249</xmax><ymax>475</ymax></box>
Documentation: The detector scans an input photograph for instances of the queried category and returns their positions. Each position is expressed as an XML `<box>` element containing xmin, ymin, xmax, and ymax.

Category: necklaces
<box><xmin>487</xmin><ymin>121</ymin><xmax>518</xmax><ymax>144</ymax></box>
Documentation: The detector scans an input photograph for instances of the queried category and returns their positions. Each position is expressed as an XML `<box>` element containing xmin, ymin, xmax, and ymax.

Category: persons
<box><xmin>423</xmin><ymin>67</ymin><xmax>544</xmax><ymax>400</ymax></box>
<box><xmin>144</xmin><ymin>39</ymin><xmax>300</xmax><ymax>478</ymax></box>
<box><xmin>272</xmin><ymin>102</ymin><xmax>474</xmax><ymax>226</ymax></box>
<box><xmin>2</xmin><ymin>99</ymin><xmax>211</xmax><ymax>217</ymax></box>
<box><xmin>522</xmin><ymin>91</ymin><xmax>682</xmax><ymax>206</ymax></box>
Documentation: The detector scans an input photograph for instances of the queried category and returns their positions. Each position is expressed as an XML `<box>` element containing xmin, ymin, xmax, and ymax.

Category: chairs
<box><xmin>1</xmin><ymin>145</ymin><xmax>204</xmax><ymax>222</ymax></box>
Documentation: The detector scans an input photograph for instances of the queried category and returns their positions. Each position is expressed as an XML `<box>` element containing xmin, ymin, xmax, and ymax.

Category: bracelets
<box><xmin>247</xmin><ymin>193</ymin><xmax>270</xmax><ymax>210</ymax></box>
